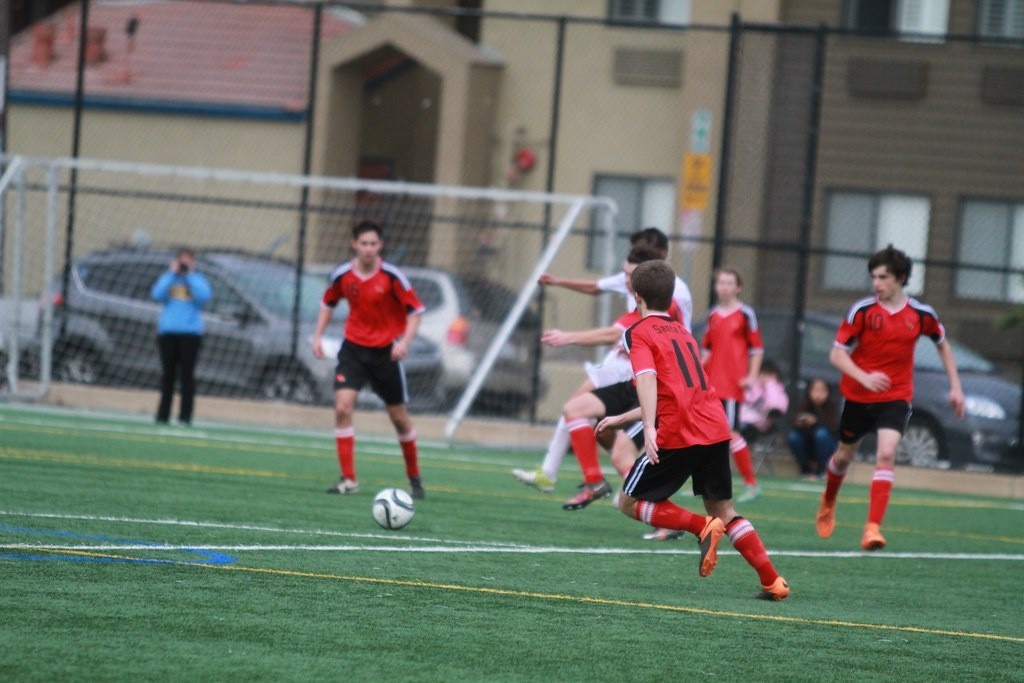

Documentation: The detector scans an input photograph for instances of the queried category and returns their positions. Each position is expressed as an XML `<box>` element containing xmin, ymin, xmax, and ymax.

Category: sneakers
<box><xmin>697</xmin><ymin>516</ymin><xmax>726</xmax><ymax>578</ymax></box>
<box><xmin>409</xmin><ymin>476</ymin><xmax>424</xmax><ymax>499</ymax></box>
<box><xmin>817</xmin><ymin>491</ymin><xmax>835</xmax><ymax>538</ymax></box>
<box><xmin>754</xmin><ymin>576</ymin><xmax>790</xmax><ymax>601</ymax></box>
<box><xmin>562</xmin><ymin>479</ymin><xmax>612</xmax><ymax>511</ymax></box>
<box><xmin>327</xmin><ymin>478</ymin><xmax>357</xmax><ymax>495</ymax></box>
<box><xmin>737</xmin><ymin>481</ymin><xmax>763</xmax><ymax>503</ymax></box>
<box><xmin>861</xmin><ymin>522</ymin><xmax>886</xmax><ymax>550</ymax></box>
<box><xmin>643</xmin><ymin>527</ymin><xmax>685</xmax><ymax>540</ymax></box>
<box><xmin>513</xmin><ymin>470</ymin><xmax>558</xmax><ymax>493</ymax></box>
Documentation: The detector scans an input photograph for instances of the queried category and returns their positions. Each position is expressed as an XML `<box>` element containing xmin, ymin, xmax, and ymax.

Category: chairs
<box><xmin>752</xmin><ymin>435</ymin><xmax>779</xmax><ymax>477</ymax></box>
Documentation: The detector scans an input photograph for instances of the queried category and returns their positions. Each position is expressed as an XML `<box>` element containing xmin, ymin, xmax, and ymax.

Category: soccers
<box><xmin>372</xmin><ymin>488</ymin><xmax>416</xmax><ymax>531</ymax></box>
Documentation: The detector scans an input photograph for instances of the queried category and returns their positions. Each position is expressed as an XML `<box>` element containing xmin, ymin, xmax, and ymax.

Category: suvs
<box><xmin>30</xmin><ymin>245</ymin><xmax>444</xmax><ymax>413</ymax></box>
<box><xmin>303</xmin><ymin>263</ymin><xmax>542</xmax><ymax>416</ymax></box>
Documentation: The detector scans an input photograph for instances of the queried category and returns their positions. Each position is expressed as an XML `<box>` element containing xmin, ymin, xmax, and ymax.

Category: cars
<box><xmin>688</xmin><ymin>310</ymin><xmax>1023</xmax><ymax>475</ymax></box>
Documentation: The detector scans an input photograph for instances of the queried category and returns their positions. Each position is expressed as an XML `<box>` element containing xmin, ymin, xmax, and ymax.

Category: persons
<box><xmin>511</xmin><ymin>227</ymin><xmax>692</xmax><ymax>494</ymax></box>
<box><xmin>814</xmin><ymin>245</ymin><xmax>965</xmax><ymax>552</ymax></box>
<box><xmin>312</xmin><ymin>220</ymin><xmax>427</xmax><ymax>504</ymax></box>
<box><xmin>620</xmin><ymin>260</ymin><xmax>791</xmax><ymax>599</ymax></box>
<box><xmin>698</xmin><ymin>266</ymin><xmax>764</xmax><ymax>502</ymax></box>
<box><xmin>538</xmin><ymin>246</ymin><xmax>689</xmax><ymax>542</ymax></box>
<box><xmin>149</xmin><ymin>242</ymin><xmax>213</xmax><ymax>433</ymax></box>
<box><xmin>737</xmin><ymin>359</ymin><xmax>842</xmax><ymax>482</ymax></box>
